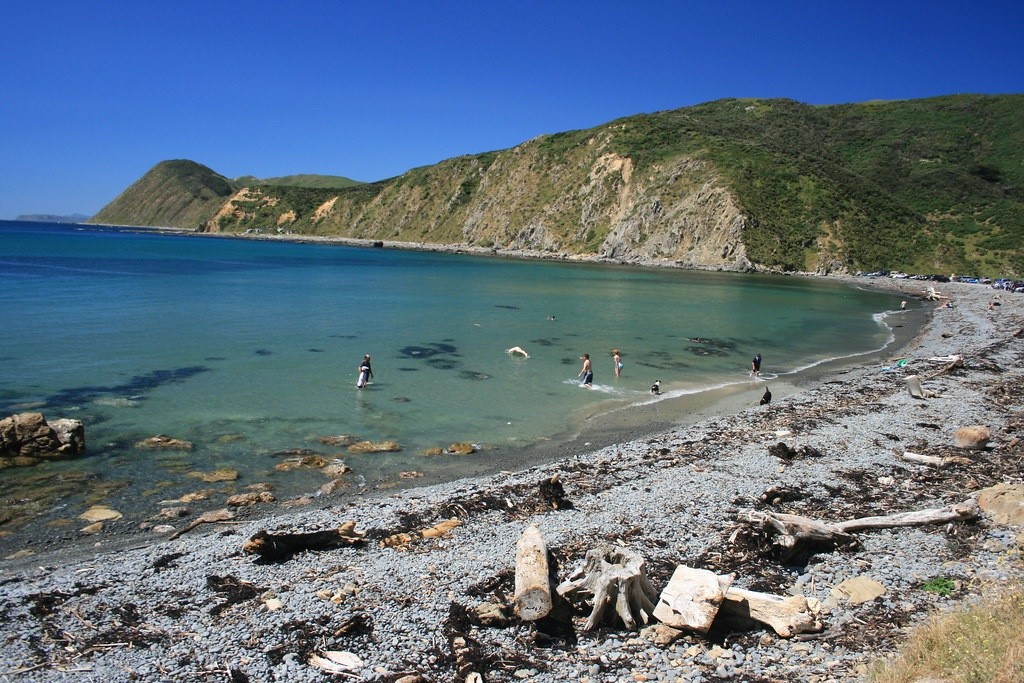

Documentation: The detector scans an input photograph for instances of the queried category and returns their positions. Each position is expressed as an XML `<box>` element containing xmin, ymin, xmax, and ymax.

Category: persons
<box><xmin>750</xmin><ymin>353</ymin><xmax>762</xmax><ymax>378</ymax></box>
<box><xmin>356</xmin><ymin>354</ymin><xmax>373</xmax><ymax>389</ymax></box>
<box><xmin>900</xmin><ymin>299</ymin><xmax>907</xmax><ymax>311</ymax></box>
<box><xmin>613</xmin><ymin>348</ymin><xmax>624</xmax><ymax>377</ymax></box>
<box><xmin>578</xmin><ymin>354</ymin><xmax>593</xmax><ymax>385</ymax></box>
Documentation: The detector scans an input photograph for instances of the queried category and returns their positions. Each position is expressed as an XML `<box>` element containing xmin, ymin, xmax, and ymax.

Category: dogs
<box><xmin>651</xmin><ymin>380</ymin><xmax>662</xmax><ymax>394</ymax></box>
<box><xmin>760</xmin><ymin>386</ymin><xmax>771</xmax><ymax>405</ymax></box>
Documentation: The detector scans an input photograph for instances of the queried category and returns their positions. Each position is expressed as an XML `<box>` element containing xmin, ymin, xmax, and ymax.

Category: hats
<box><xmin>362</xmin><ymin>365</ymin><xmax>369</xmax><ymax>370</ymax></box>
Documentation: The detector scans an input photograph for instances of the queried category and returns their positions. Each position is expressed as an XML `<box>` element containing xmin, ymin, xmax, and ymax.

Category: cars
<box><xmin>959</xmin><ymin>275</ymin><xmax>992</xmax><ymax>285</ymax></box>
<box><xmin>993</xmin><ymin>278</ymin><xmax>1024</xmax><ymax>293</ymax></box>
<box><xmin>862</xmin><ymin>269</ymin><xmax>951</xmax><ymax>283</ymax></box>
